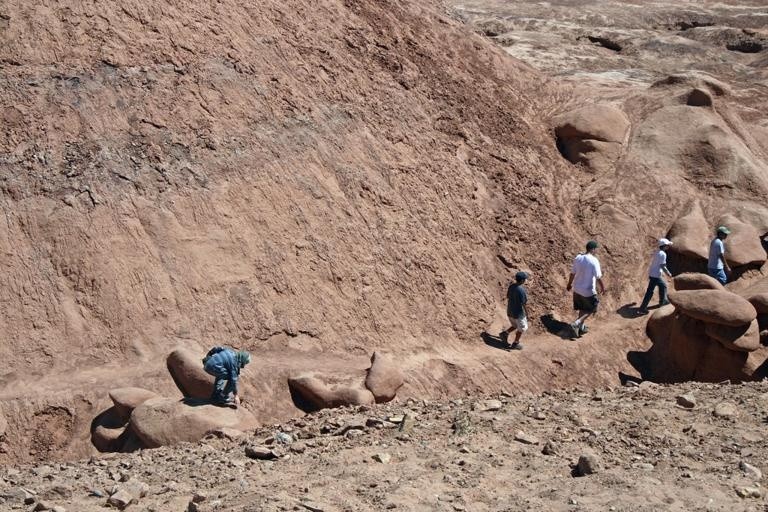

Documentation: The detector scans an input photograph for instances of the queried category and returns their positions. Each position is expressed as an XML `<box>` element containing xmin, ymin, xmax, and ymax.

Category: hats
<box><xmin>515</xmin><ymin>272</ymin><xmax>528</xmax><ymax>279</ymax></box>
<box><xmin>586</xmin><ymin>240</ymin><xmax>600</xmax><ymax>248</ymax></box>
<box><xmin>657</xmin><ymin>238</ymin><xmax>673</xmax><ymax>247</ymax></box>
<box><xmin>717</xmin><ymin>227</ymin><xmax>730</xmax><ymax>236</ymax></box>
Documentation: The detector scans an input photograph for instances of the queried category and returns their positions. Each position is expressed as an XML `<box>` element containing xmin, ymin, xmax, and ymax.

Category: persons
<box><xmin>202</xmin><ymin>345</ymin><xmax>251</xmax><ymax>406</ymax></box>
<box><xmin>707</xmin><ymin>225</ymin><xmax>733</xmax><ymax>286</ymax></box>
<box><xmin>567</xmin><ymin>241</ymin><xmax>606</xmax><ymax>339</ymax></box>
<box><xmin>499</xmin><ymin>271</ymin><xmax>532</xmax><ymax>350</ymax></box>
<box><xmin>637</xmin><ymin>237</ymin><xmax>673</xmax><ymax>315</ymax></box>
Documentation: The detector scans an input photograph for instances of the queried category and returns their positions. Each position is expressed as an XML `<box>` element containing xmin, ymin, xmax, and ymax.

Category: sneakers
<box><xmin>570</xmin><ymin>322</ymin><xmax>588</xmax><ymax>338</ymax></box>
<box><xmin>511</xmin><ymin>342</ymin><xmax>523</xmax><ymax>349</ymax></box>
<box><xmin>660</xmin><ymin>300</ymin><xmax>669</xmax><ymax>306</ymax></box>
<box><xmin>499</xmin><ymin>331</ymin><xmax>509</xmax><ymax>345</ymax></box>
<box><xmin>637</xmin><ymin>308</ymin><xmax>649</xmax><ymax>315</ymax></box>
